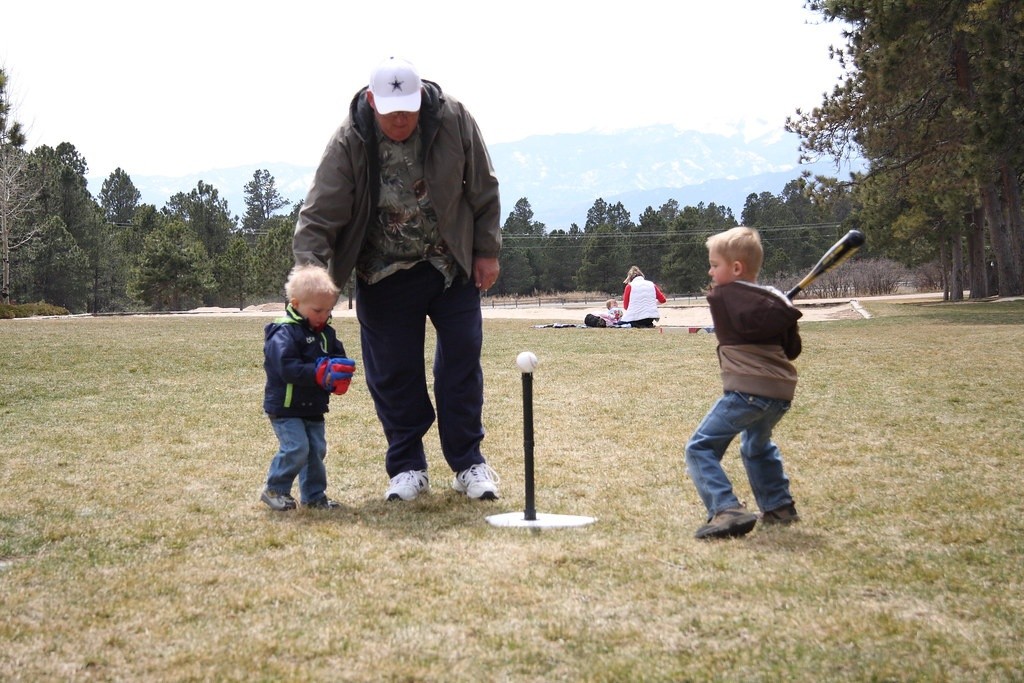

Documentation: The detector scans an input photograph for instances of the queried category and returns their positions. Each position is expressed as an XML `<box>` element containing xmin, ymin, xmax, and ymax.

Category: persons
<box><xmin>599</xmin><ymin>299</ymin><xmax>623</xmax><ymax>327</ymax></box>
<box><xmin>621</xmin><ymin>267</ymin><xmax>666</xmax><ymax>329</ymax></box>
<box><xmin>293</xmin><ymin>56</ymin><xmax>501</xmax><ymax>499</ymax></box>
<box><xmin>260</xmin><ymin>264</ymin><xmax>348</xmax><ymax>511</ymax></box>
<box><xmin>686</xmin><ymin>228</ymin><xmax>803</xmax><ymax>538</ymax></box>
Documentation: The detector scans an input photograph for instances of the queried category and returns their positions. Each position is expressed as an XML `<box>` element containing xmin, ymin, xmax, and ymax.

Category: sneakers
<box><xmin>260</xmin><ymin>484</ymin><xmax>297</xmax><ymax>512</ymax></box>
<box><xmin>384</xmin><ymin>467</ymin><xmax>430</xmax><ymax>501</ymax></box>
<box><xmin>452</xmin><ymin>462</ymin><xmax>501</xmax><ymax>499</ymax></box>
<box><xmin>756</xmin><ymin>501</ymin><xmax>801</xmax><ymax>532</ymax></box>
<box><xmin>299</xmin><ymin>497</ymin><xmax>344</xmax><ymax>513</ymax></box>
<box><xmin>695</xmin><ymin>500</ymin><xmax>757</xmax><ymax>538</ymax></box>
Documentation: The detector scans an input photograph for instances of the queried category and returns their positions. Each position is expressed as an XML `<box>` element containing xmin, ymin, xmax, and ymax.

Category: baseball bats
<box><xmin>786</xmin><ymin>229</ymin><xmax>866</xmax><ymax>300</ymax></box>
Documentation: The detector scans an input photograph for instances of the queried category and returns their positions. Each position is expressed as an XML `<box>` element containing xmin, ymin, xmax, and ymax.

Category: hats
<box><xmin>369</xmin><ymin>56</ymin><xmax>422</xmax><ymax>115</ymax></box>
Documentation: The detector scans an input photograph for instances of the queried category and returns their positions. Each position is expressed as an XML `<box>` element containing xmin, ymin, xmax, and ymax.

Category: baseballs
<box><xmin>516</xmin><ymin>351</ymin><xmax>537</xmax><ymax>373</ymax></box>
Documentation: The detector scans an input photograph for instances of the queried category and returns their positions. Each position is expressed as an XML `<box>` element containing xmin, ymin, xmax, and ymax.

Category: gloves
<box><xmin>314</xmin><ymin>356</ymin><xmax>356</xmax><ymax>395</ymax></box>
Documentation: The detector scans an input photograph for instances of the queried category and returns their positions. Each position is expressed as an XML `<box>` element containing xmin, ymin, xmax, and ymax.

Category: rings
<box><xmin>491</xmin><ymin>281</ymin><xmax>495</xmax><ymax>284</ymax></box>
